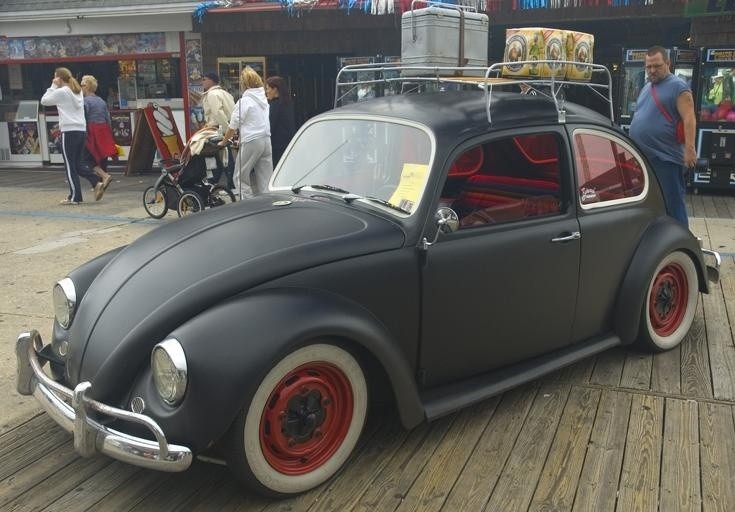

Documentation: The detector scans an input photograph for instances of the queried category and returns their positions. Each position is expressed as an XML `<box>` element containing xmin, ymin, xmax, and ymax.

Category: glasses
<box><xmin>81</xmin><ymin>85</ymin><xmax>85</xmax><ymax>87</ymax></box>
<box><xmin>202</xmin><ymin>78</ymin><xmax>208</xmax><ymax>81</ymax></box>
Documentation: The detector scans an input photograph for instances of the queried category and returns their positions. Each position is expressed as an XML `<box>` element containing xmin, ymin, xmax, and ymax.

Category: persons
<box><xmin>357</xmin><ymin>79</ymin><xmax>375</xmax><ymax>101</ymax></box>
<box><xmin>201</xmin><ymin>66</ymin><xmax>295</xmax><ymax>198</ymax></box>
<box><xmin>41</xmin><ymin>67</ymin><xmax>118</xmax><ymax>205</ymax></box>
<box><xmin>629</xmin><ymin>45</ymin><xmax>697</xmax><ymax>229</ymax></box>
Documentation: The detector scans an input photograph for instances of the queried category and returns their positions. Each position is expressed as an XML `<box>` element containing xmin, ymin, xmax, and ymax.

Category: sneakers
<box><xmin>94</xmin><ymin>175</ymin><xmax>112</xmax><ymax>201</ymax></box>
<box><xmin>60</xmin><ymin>197</ymin><xmax>80</xmax><ymax>205</ymax></box>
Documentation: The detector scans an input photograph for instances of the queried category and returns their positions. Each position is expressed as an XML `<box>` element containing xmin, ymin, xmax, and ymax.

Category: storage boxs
<box><xmin>401</xmin><ymin>0</ymin><xmax>489</xmax><ymax>76</ymax></box>
<box><xmin>502</xmin><ymin>27</ymin><xmax>595</xmax><ymax>81</ymax></box>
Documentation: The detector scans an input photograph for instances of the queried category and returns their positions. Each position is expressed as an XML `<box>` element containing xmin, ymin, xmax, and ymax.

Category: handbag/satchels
<box><xmin>676</xmin><ymin>121</ymin><xmax>685</xmax><ymax>144</ymax></box>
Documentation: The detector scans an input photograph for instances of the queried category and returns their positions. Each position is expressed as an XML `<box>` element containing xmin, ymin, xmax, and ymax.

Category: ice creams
<box><xmin>152</xmin><ymin>101</ymin><xmax>182</xmax><ymax>158</ymax></box>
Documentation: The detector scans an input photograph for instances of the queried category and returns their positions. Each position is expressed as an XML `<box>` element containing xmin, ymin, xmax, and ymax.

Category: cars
<box><xmin>15</xmin><ymin>60</ymin><xmax>721</xmax><ymax>501</ymax></box>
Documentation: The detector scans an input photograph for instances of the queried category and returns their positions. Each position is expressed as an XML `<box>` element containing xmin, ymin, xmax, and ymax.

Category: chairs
<box><xmin>446</xmin><ymin>175</ymin><xmax>561</xmax><ymax>226</ymax></box>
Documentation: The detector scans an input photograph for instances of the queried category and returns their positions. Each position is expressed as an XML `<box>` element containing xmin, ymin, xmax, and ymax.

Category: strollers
<box><xmin>143</xmin><ymin>135</ymin><xmax>236</xmax><ymax>219</ymax></box>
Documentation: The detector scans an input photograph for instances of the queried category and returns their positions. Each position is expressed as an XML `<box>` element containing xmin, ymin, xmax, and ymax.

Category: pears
<box><xmin>509</xmin><ymin>44</ymin><xmax>520</xmax><ymax>62</ymax></box>
<box><xmin>550</xmin><ymin>44</ymin><xmax>559</xmax><ymax>58</ymax></box>
<box><xmin>578</xmin><ymin>47</ymin><xmax>585</xmax><ymax>60</ymax></box>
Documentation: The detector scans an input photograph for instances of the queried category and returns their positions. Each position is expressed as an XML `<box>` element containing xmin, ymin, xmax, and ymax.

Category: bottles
<box><xmin>217</xmin><ymin>124</ymin><xmax>224</xmax><ymax>140</ymax></box>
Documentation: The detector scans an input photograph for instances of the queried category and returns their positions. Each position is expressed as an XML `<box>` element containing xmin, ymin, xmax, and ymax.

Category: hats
<box><xmin>201</xmin><ymin>71</ymin><xmax>219</xmax><ymax>83</ymax></box>
